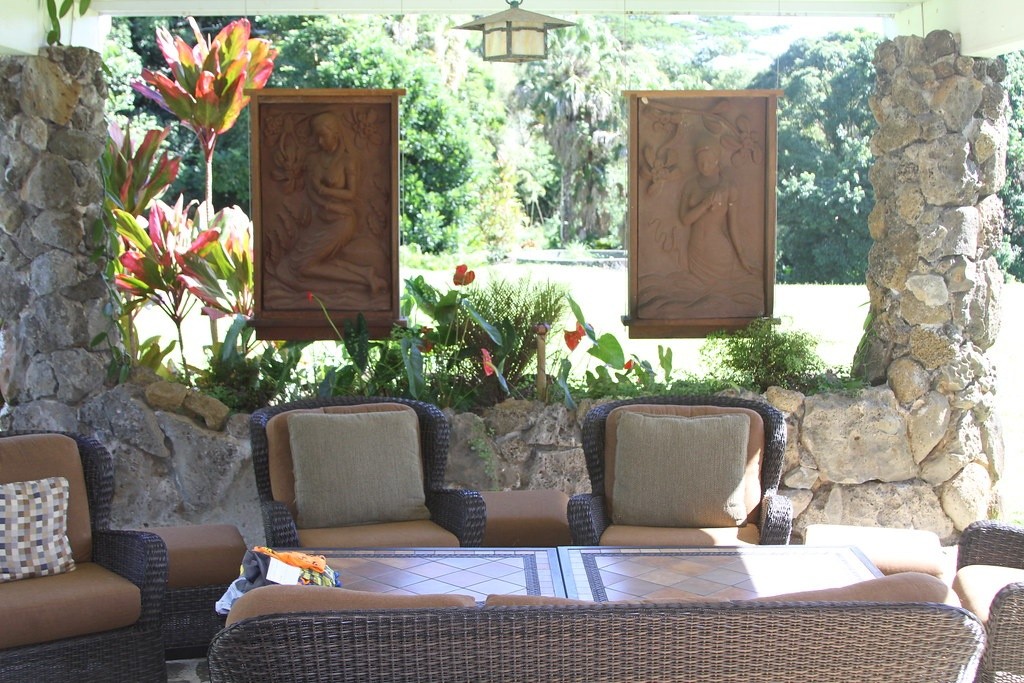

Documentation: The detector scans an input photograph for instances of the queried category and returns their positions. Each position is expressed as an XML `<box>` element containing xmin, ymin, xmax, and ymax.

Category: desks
<box><xmin>247</xmin><ymin>545</ymin><xmax>887</xmax><ymax>604</ymax></box>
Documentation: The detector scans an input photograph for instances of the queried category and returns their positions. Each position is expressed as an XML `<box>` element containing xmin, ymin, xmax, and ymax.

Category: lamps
<box><xmin>450</xmin><ymin>0</ymin><xmax>579</xmax><ymax>64</ymax></box>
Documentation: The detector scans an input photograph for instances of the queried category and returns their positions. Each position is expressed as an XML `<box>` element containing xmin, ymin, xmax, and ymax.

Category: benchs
<box><xmin>203</xmin><ymin>581</ymin><xmax>987</xmax><ymax>683</ymax></box>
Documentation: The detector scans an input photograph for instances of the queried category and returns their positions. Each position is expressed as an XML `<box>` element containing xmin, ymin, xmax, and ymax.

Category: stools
<box><xmin>799</xmin><ymin>522</ymin><xmax>945</xmax><ymax>587</ymax></box>
<box><xmin>478</xmin><ymin>489</ymin><xmax>571</xmax><ymax>549</ymax></box>
<box><xmin>124</xmin><ymin>526</ymin><xmax>244</xmax><ymax>656</ymax></box>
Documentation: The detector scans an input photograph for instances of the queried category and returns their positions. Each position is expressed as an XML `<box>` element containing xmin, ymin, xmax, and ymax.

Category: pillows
<box><xmin>285</xmin><ymin>409</ymin><xmax>436</xmax><ymax>527</ymax></box>
<box><xmin>612</xmin><ymin>411</ymin><xmax>749</xmax><ymax>529</ymax></box>
<box><xmin>0</xmin><ymin>474</ymin><xmax>76</xmax><ymax>584</ymax></box>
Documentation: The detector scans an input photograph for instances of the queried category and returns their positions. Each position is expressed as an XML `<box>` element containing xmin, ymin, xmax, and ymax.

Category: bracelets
<box><xmin>318</xmin><ymin>186</ymin><xmax>323</xmax><ymax>194</ymax></box>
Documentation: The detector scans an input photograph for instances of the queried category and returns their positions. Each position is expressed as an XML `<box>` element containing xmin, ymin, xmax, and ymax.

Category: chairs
<box><xmin>0</xmin><ymin>431</ymin><xmax>167</xmax><ymax>683</ymax></box>
<box><xmin>558</xmin><ymin>391</ymin><xmax>793</xmax><ymax>550</ymax></box>
<box><xmin>951</xmin><ymin>517</ymin><xmax>1024</xmax><ymax>683</ymax></box>
<box><xmin>247</xmin><ymin>395</ymin><xmax>485</xmax><ymax>552</ymax></box>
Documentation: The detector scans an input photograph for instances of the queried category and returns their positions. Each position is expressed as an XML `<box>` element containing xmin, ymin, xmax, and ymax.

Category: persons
<box><xmin>289</xmin><ymin>111</ymin><xmax>388</xmax><ymax>296</ymax></box>
<box><xmin>679</xmin><ymin>136</ymin><xmax>754</xmax><ymax>284</ymax></box>
